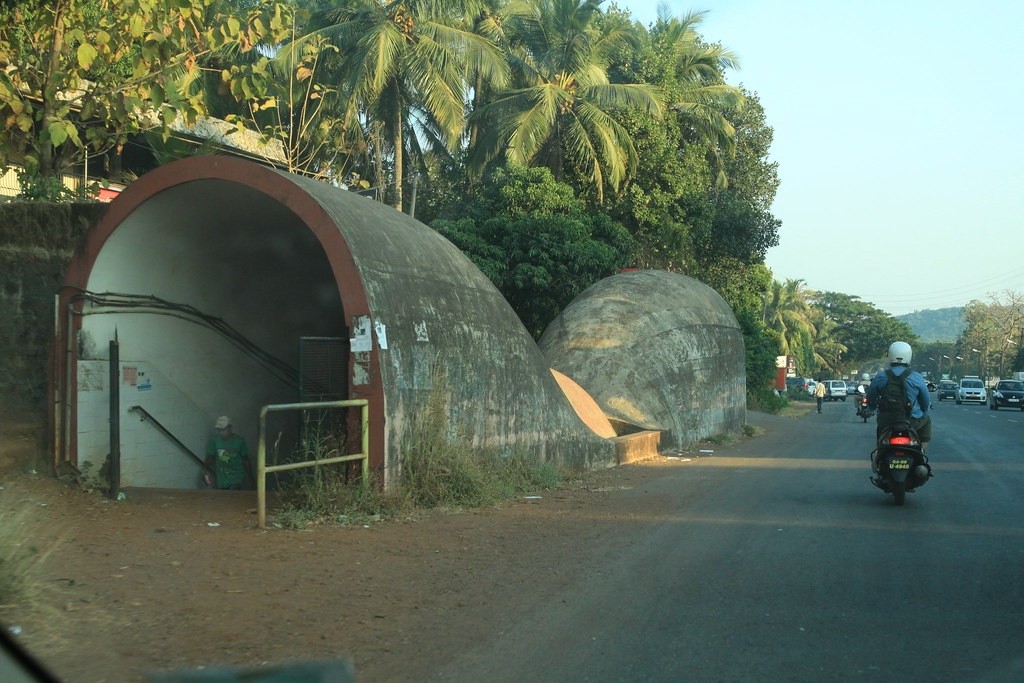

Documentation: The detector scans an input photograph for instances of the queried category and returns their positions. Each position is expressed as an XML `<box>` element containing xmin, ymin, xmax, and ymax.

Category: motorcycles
<box><xmin>855</xmin><ymin>389</ymin><xmax>874</xmax><ymax>422</ymax></box>
<box><xmin>869</xmin><ymin>418</ymin><xmax>934</xmax><ymax>506</ymax></box>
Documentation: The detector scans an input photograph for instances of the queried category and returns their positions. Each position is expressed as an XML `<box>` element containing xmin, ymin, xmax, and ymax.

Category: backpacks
<box><xmin>877</xmin><ymin>369</ymin><xmax>914</xmax><ymax>425</ymax></box>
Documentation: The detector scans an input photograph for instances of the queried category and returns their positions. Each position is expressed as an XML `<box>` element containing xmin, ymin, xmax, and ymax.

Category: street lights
<box><xmin>956</xmin><ymin>357</ymin><xmax>968</xmax><ymax>376</ymax></box>
<box><xmin>929</xmin><ymin>356</ymin><xmax>942</xmax><ymax>373</ymax></box>
<box><xmin>942</xmin><ymin>355</ymin><xmax>953</xmax><ymax>377</ymax></box>
<box><xmin>972</xmin><ymin>345</ymin><xmax>989</xmax><ymax>380</ymax></box>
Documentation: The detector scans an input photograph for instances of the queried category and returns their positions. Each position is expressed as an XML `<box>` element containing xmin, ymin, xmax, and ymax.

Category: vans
<box><xmin>822</xmin><ymin>380</ymin><xmax>847</xmax><ymax>402</ymax></box>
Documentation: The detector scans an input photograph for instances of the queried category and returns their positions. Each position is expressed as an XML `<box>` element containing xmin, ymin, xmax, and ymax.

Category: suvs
<box><xmin>954</xmin><ymin>375</ymin><xmax>987</xmax><ymax>406</ymax></box>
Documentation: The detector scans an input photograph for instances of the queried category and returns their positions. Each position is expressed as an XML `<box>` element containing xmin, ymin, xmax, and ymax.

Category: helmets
<box><xmin>889</xmin><ymin>341</ymin><xmax>913</xmax><ymax>366</ymax></box>
<box><xmin>863</xmin><ymin>373</ymin><xmax>870</xmax><ymax>381</ymax></box>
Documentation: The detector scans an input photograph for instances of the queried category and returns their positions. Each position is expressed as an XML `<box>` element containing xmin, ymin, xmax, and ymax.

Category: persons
<box><xmin>853</xmin><ymin>373</ymin><xmax>873</xmax><ymax>415</ymax></box>
<box><xmin>202</xmin><ymin>416</ymin><xmax>254</xmax><ymax>490</ymax></box>
<box><xmin>866</xmin><ymin>341</ymin><xmax>931</xmax><ymax>449</ymax></box>
<box><xmin>813</xmin><ymin>378</ymin><xmax>826</xmax><ymax>414</ymax></box>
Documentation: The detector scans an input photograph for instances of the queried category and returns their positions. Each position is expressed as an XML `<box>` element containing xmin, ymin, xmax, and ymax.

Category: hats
<box><xmin>215</xmin><ymin>416</ymin><xmax>232</xmax><ymax>428</ymax></box>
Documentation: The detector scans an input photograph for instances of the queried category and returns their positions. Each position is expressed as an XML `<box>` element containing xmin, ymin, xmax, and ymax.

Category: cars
<box><xmin>989</xmin><ymin>380</ymin><xmax>1024</xmax><ymax>411</ymax></box>
<box><xmin>937</xmin><ymin>379</ymin><xmax>960</xmax><ymax>401</ymax></box>
<box><xmin>845</xmin><ymin>381</ymin><xmax>861</xmax><ymax>395</ymax></box>
